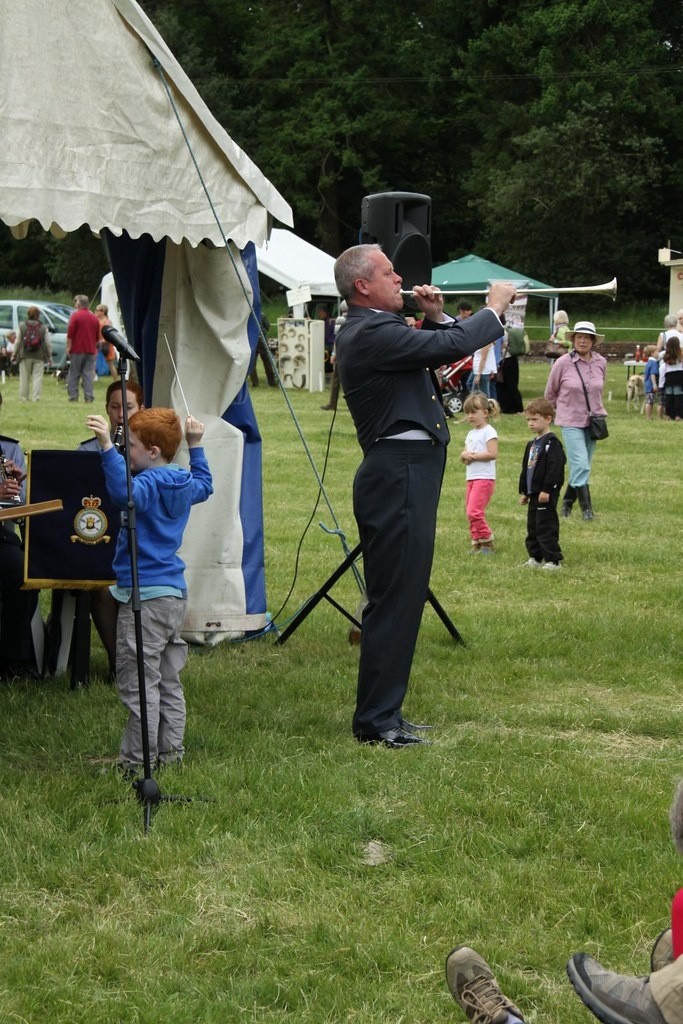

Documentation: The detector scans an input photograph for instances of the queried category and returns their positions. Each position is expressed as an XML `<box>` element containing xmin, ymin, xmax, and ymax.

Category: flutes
<box><xmin>0</xmin><ymin>444</ymin><xmax>25</xmax><ymax>551</ymax></box>
<box><xmin>113</xmin><ymin>423</ymin><xmax>124</xmax><ymax>449</ymax></box>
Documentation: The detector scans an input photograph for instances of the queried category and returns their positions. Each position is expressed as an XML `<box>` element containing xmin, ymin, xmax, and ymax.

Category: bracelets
<box><xmin>554</xmin><ymin>339</ymin><xmax>558</xmax><ymax>344</ymax></box>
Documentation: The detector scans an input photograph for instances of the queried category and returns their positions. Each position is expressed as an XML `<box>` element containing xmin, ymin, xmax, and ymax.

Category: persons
<box><xmin>445</xmin><ymin>927</ymin><xmax>683</xmax><ymax>1024</ymax></box>
<box><xmin>248</xmin><ymin>300</ymin><xmax>349</xmax><ymax>410</ymax></box>
<box><xmin>332</xmin><ymin>245</ymin><xmax>517</xmax><ymax>750</ymax></box>
<box><xmin>644</xmin><ymin>309</ymin><xmax>683</xmax><ymax>420</ymax></box>
<box><xmin>518</xmin><ymin>398</ymin><xmax>567</xmax><ymax>570</ymax></box>
<box><xmin>0</xmin><ymin>294</ymin><xmax>214</xmax><ymax>769</ymax></box>
<box><xmin>543</xmin><ymin>321</ymin><xmax>609</xmax><ymax>521</ymax></box>
<box><xmin>459</xmin><ymin>390</ymin><xmax>499</xmax><ymax>556</ymax></box>
<box><xmin>404</xmin><ymin>301</ymin><xmax>573</xmax><ymax>414</ymax></box>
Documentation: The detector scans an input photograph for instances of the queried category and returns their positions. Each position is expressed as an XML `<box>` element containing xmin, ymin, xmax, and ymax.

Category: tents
<box><xmin>0</xmin><ymin>1</ymin><xmax>295</xmax><ymax>645</ymax></box>
<box><xmin>102</xmin><ymin>225</ymin><xmax>342</xmax><ymax>385</ymax></box>
<box><xmin>432</xmin><ymin>254</ymin><xmax>558</xmax><ymax>339</ymax></box>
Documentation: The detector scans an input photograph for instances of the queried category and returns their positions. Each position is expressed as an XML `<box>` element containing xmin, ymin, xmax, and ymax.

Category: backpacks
<box><xmin>23</xmin><ymin>321</ymin><xmax>43</xmax><ymax>352</ymax></box>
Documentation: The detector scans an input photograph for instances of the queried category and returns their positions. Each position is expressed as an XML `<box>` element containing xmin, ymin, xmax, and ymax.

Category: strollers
<box><xmin>437</xmin><ymin>355</ymin><xmax>473</xmax><ymax>414</ymax></box>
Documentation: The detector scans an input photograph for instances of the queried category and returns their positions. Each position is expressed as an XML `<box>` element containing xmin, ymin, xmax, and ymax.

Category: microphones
<box><xmin>100</xmin><ymin>325</ymin><xmax>140</xmax><ymax>362</ymax></box>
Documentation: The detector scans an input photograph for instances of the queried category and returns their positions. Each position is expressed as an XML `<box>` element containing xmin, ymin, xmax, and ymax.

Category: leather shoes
<box><xmin>358</xmin><ymin>728</ymin><xmax>432</xmax><ymax>749</ymax></box>
<box><xmin>400</xmin><ymin>719</ymin><xmax>433</xmax><ymax>733</ymax></box>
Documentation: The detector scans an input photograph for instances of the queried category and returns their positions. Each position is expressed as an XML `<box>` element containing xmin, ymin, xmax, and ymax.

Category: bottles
<box><xmin>636</xmin><ymin>345</ymin><xmax>640</xmax><ymax>362</ymax></box>
<box><xmin>643</xmin><ymin>348</ymin><xmax>648</xmax><ymax>362</ymax></box>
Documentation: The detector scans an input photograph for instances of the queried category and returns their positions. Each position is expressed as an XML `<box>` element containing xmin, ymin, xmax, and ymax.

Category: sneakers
<box><xmin>567</xmin><ymin>952</ymin><xmax>667</xmax><ymax>1024</ymax></box>
<box><xmin>445</xmin><ymin>946</ymin><xmax>525</xmax><ymax>1024</ymax></box>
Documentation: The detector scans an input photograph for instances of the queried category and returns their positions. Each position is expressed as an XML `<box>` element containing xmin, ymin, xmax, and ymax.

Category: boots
<box><xmin>576</xmin><ymin>484</ymin><xmax>594</xmax><ymax>522</ymax></box>
<box><xmin>467</xmin><ymin>534</ymin><xmax>497</xmax><ymax>558</ymax></box>
<box><xmin>69</xmin><ymin>618</ymin><xmax>91</xmax><ymax>692</ymax></box>
<box><xmin>95</xmin><ymin>622</ymin><xmax>119</xmax><ymax>686</ymax></box>
<box><xmin>561</xmin><ymin>483</ymin><xmax>577</xmax><ymax>519</ymax></box>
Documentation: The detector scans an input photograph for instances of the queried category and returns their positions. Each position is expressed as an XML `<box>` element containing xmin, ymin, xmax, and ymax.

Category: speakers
<box><xmin>361</xmin><ymin>192</ymin><xmax>434</xmax><ymax>314</ymax></box>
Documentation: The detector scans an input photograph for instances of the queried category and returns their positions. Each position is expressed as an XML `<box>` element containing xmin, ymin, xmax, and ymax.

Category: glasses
<box><xmin>93</xmin><ymin>309</ymin><xmax>103</xmax><ymax>312</ymax></box>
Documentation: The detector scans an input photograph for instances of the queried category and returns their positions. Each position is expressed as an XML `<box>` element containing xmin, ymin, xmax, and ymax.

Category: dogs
<box><xmin>626</xmin><ymin>375</ymin><xmax>645</xmax><ymax>411</ymax></box>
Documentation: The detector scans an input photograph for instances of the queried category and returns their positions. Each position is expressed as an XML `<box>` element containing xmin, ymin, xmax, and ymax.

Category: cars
<box><xmin>0</xmin><ymin>299</ymin><xmax>78</xmax><ymax>370</ymax></box>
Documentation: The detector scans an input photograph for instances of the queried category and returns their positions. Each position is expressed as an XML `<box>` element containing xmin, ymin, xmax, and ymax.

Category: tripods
<box><xmin>103</xmin><ymin>352</ymin><xmax>218</xmax><ymax>835</ymax></box>
<box><xmin>274</xmin><ymin>546</ymin><xmax>460</xmax><ymax>646</ymax></box>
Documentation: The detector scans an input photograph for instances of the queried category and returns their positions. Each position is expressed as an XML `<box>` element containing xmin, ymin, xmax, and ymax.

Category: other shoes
<box><xmin>269</xmin><ymin>382</ymin><xmax>278</xmax><ymax>387</ymax></box>
<box><xmin>649</xmin><ymin>928</ymin><xmax>675</xmax><ymax>972</ymax></box>
<box><xmin>320</xmin><ymin>404</ymin><xmax>337</xmax><ymax>411</ymax></box>
<box><xmin>69</xmin><ymin>397</ymin><xmax>79</xmax><ymax>403</ymax></box>
<box><xmin>0</xmin><ymin>660</ymin><xmax>43</xmax><ymax>683</ymax></box>
<box><xmin>522</xmin><ymin>557</ymin><xmax>545</xmax><ymax>570</ymax></box>
<box><xmin>542</xmin><ymin>560</ymin><xmax>563</xmax><ymax>571</ymax></box>
<box><xmin>251</xmin><ymin>383</ymin><xmax>259</xmax><ymax>388</ymax></box>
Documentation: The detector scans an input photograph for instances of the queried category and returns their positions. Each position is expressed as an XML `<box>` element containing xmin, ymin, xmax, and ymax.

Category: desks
<box><xmin>624</xmin><ymin>359</ymin><xmax>648</xmax><ymax>401</ymax></box>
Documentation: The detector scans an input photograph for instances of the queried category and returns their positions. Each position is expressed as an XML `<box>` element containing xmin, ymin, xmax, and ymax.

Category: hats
<box><xmin>564</xmin><ymin>321</ymin><xmax>606</xmax><ymax>347</ymax></box>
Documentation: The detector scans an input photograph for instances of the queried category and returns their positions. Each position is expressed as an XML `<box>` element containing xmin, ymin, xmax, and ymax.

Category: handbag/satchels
<box><xmin>590</xmin><ymin>415</ymin><xmax>609</xmax><ymax>441</ymax></box>
<box><xmin>657</xmin><ymin>332</ymin><xmax>667</xmax><ymax>351</ymax></box>
